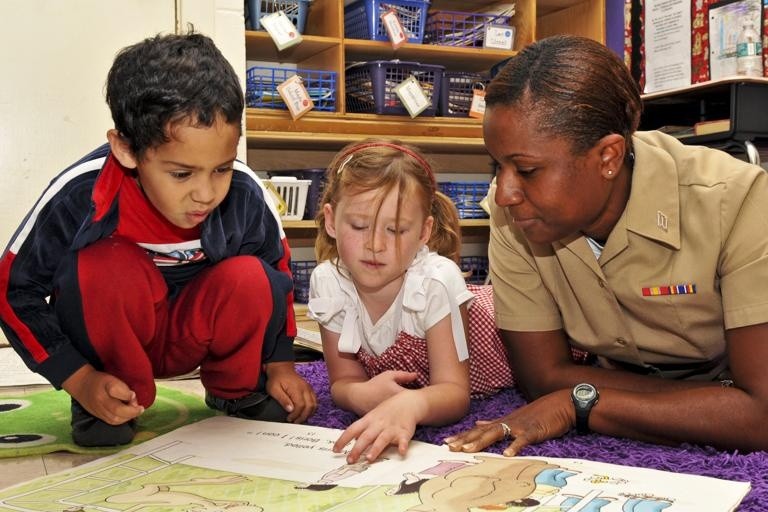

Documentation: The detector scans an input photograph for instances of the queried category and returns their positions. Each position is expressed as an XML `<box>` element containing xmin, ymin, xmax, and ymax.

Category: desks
<box><xmin>634</xmin><ymin>75</ymin><xmax>767</xmax><ymax>171</ymax></box>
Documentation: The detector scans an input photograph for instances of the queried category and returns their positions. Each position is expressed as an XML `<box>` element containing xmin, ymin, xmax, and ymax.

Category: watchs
<box><xmin>571</xmin><ymin>383</ymin><xmax>598</xmax><ymax>434</ymax></box>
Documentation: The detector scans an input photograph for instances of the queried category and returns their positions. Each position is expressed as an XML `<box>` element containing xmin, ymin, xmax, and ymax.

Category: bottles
<box><xmin>734</xmin><ymin>17</ymin><xmax>763</xmax><ymax>78</ymax></box>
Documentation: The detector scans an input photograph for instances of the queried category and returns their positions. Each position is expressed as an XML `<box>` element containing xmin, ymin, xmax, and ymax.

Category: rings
<box><xmin>498</xmin><ymin>422</ymin><xmax>512</xmax><ymax>441</ymax></box>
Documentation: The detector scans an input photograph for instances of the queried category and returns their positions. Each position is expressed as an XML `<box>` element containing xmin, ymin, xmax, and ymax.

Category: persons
<box><xmin>0</xmin><ymin>30</ymin><xmax>321</xmax><ymax>448</ymax></box>
<box><xmin>441</xmin><ymin>32</ymin><xmax>768</xmax><ymax>458</ymax></box>
<box><xmin>307</xmin><ymin>137</ymin><xmax>515</xmax><ymax>465</ymax></box>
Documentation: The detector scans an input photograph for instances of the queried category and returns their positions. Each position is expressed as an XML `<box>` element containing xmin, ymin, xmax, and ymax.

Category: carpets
<box><xmin>290</xmin><ymin>361</ymin><xmax>767</xmax><ymax>512</ymax></box>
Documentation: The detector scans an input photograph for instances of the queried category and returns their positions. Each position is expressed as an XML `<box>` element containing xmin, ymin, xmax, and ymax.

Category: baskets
<box><xmin>344</xmin><ymin>62</ymin><xmax>491</xmax><ymax>116</ymax></box>
<box><xmin>258</xmin><ymin>168</ymin><xmax>332</xmax><ymax>220</ymax></box>
<box><xmin>248</xmin><ymin>0</ymin><xmax>311</xmax><ymax>35</ymax></box>
<box><xmin>423</xmin><ymin>10</ymin><xmax>511</xmax><ymax>48</ymax></box>
<box><xmin>437</xmin><ymin>181</ymin><xmax>491</xmax><ymax>219</ymax></box>
<box><xmin>344</xmin><ymin>0</ymin><xmax>432</xmax><ymax>44</ymax></box>
<box><xmin>291</xmin><ymin>260</ymin><xmax>318</xmax><ymax>304</ymax></box>
<box><xmin>247</xmin><ymin>65</ymin><xmax>338</xmax><ymax>112</ymax></box>
<box><xmin>457</xmin><ymin>256</ymin><xmax>490</xmax><ymax>285</ymax></box>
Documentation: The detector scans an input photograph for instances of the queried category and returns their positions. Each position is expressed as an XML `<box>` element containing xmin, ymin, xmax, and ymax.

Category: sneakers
<box><xmin>205</xmin><ymin>387</ymin><xmax>291</xmax><ymax>422</ymax></box>
<box><xmin>70</xmin><ymin>394</ymin><xmax>135</xmax><ymax>446</ymax></box>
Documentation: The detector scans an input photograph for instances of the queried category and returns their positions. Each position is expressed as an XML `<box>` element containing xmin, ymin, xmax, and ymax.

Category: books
<box><xmin>247</xmin><ymin>2</ymin><xmax>520</xmax><ymax>224</ymax></box>
<box><xmin>1</xmin><ymin>411</ymin><xmax>751</xmax><ymax>512</ymax></box>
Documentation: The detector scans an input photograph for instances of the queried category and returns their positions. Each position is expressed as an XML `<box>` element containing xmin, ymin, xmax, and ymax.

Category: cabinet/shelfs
<box><xmin>243</xmin><ymin>1</ymin><xmax>605</xmax><ymax>317</ymax></box>
